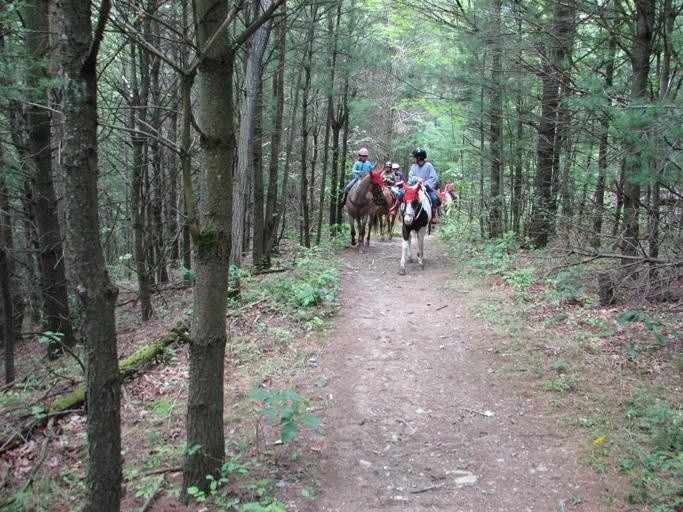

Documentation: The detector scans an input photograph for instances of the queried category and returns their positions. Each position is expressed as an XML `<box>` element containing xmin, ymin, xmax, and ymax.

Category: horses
<box><xmin>399</xmin><ymin>180</ymin><xmax>432</xmax><ymax>274</ymax></box>
<box><xmin>438</xmin><ymin>192</ymin><xmax>459</xmax><ymax>217</ymax></box>
<box><xmin>345</xmin><ymin>166</ymin><xmax>404</xmax><ymax>253</ymax></box>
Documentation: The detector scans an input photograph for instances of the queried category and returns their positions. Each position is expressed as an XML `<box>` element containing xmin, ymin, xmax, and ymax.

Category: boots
<box><xmin>432</xmin><ymin>208</ymin><xmax>437</xmax><ymax>224</ymax></box>
<box><xmin>389</xmin><ymin>201</ymin><xmax>401</xmax><ymax>212</ymax></box>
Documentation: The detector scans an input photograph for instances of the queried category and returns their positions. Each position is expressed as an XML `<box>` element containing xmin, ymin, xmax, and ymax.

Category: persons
<box><xmin>379</xmin><ymin>161</ymin><xmax>453</xmax><ymax>215</ymax></box>
<box><xmin>387</xmin><ymin>147</ymin><xmax>439</xmax><ymax>224</ymax></box>
<box><xmin>339</xmin><ymin>147</ymin><xmax>373</xmax><ymax>208</ymax></box>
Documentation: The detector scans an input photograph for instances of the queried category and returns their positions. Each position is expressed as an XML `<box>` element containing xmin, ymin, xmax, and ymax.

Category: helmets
<box><xmin>359</xmin><ymin>148</ymin><xmax>368</xmax><ymax>155</ymax></box>
<box><xmin>410</xmin><ymin>148</ymin><xmax>426</xmax><ymax>159</ymax></box>
<box><xmin>385</xmin><ymin>161</ymin><xmax>391</xmax><ymax>167</ymax></box>
<box><xmin>392</xmin><ymin>163</ymin><xmax>399</xmax><ymax>169</ymax></box>
